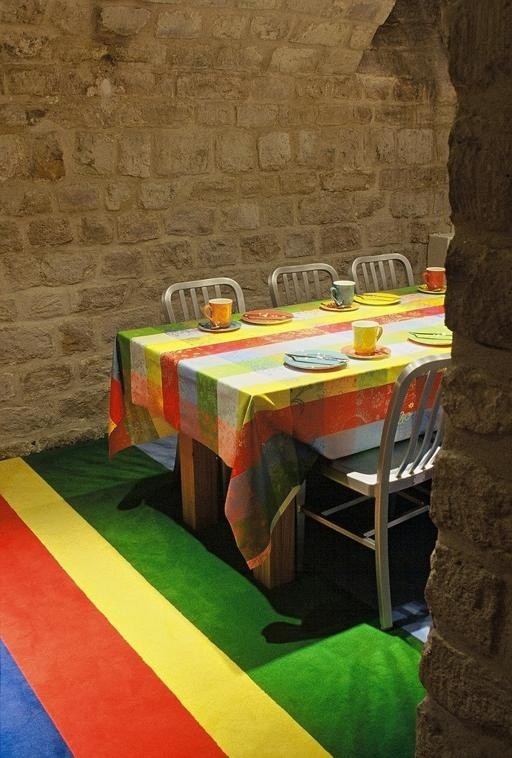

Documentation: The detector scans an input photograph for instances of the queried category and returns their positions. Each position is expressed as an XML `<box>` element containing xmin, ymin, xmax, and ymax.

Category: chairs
<box><xmin>270</xmin><ymin>263</ymin><xmax>340</xmax><ymax>307</ymax></box>
<box><xmin>351</xmin><ymin>253</ymin><xmax>416</xmax><ymax>296</ymax></box>
<box><xmin>299</xmin><ymin>354</ymin><xmax>452</xmax><ymax>630</ymax></box>
<box><xmin>160</xmin><ymin>276</ymin><xmax>248</xmax><ymax>503</ymax></box>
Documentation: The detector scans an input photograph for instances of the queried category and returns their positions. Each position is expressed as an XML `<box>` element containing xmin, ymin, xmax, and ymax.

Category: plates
<box><xmin>240</xmin><ymin>309</ymin><xmax>294</xmax><ymax>325</ymax></box>
<box><xmin>198</xmin><ymin>320</ymin><xmax>241</xmax><ymax>332</ymax></box>
<box><xmin>320</xmin><ymin>302</ymin><xmax>358</xmax><ymax>311</ymax></box>
<box><xmin>353</xmin><ymin>292</ymin><xmax>401</xmax><ymax>305</ymax></box>
<box><xmin>407</xmin><ymin>326</ymin><xmax>452</xmax><ymax>346</ymax></box>
<box><xmin>285</xmin><ymin>349</ymin><xmax>346</xmax><ymax>370</ymax></box>
<box><xmin>417</xmin><ymin>284</ymin><xmax>446</xmax><ymax>294</ymax></box>
<box><xmin>340</xmin><ymin>344</ymin><xmax>390</xmax><ymax>359</ymax></box>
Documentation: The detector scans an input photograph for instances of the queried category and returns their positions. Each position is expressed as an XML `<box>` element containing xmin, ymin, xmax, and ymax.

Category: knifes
<box><xmin>286</xmin><ymin>352</ymin><xmax>348</xmax><ymax>361</ymax></box>
<box><xmin>408</xmin><ymin>331</ymin><xmax>452</xmax><ymax>336</ymax></box>
<box><xmin>243</xmin><ymin>313</ymin><xmax>289</xmax><ymax>318</ymax></box>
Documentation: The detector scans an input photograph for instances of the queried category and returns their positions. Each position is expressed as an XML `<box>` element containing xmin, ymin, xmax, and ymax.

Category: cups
<box><xmin>330</xmin><ymin>280</ymin><xmax>356</xmax><ymax>307</ymax></box>
<box><xmin>422</xmin><ymin>267</ymin><xmax>445</xmax><ymax>291</ymax></box>
<box><xmin>202</xmin><ymin>298</ymin><xmax>232</xmax><ymax>328</ymax></box>
<box><xmin>351</xmin><ymin>320</ymin><xmax>383</xmax><ymax>354</ymax></box>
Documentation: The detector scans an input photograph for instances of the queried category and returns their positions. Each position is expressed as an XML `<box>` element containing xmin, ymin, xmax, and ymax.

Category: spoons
<box><xmin>200</xmin><ymin>323</ymin><xmax>220</xmax><ymax>330</ymax></box>
